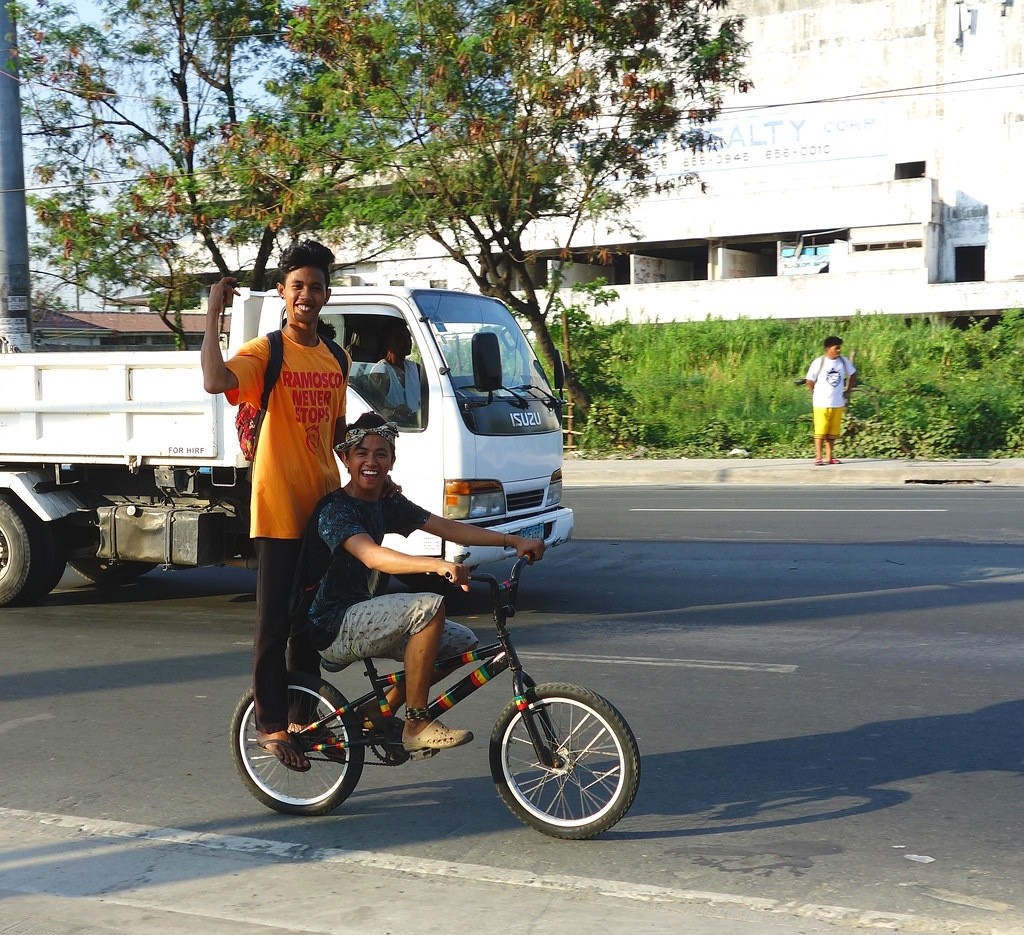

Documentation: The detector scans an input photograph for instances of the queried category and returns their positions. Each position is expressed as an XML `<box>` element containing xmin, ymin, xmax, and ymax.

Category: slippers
<box><xmin>814</xmin><ymin>459</ymin><xmax>823</xmax><ymax>465</ymax></box>
<box><xmin>404</xmin><ymin>719</ymin><xmax>474</xmax><ymax>751</ymax></box>
<box><xmin>245</xmin><ymin>739</ymin><xmax>313</xmax><ymax>771</ymax></box>
<box><xmin>826</xmin><ymin>459</ymin><xmax>840</xmax><ymax>464</ymax></box>
<box><xmin>307</xmin><ymin>729</ymin><xmax>347</xmax><ymax>761</ymax></box>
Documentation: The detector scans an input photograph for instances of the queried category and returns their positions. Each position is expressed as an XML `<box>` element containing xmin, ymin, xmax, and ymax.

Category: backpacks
<box><xmin>237</xmin><ymin>329</ymin><xmax>350</xmax><ymax>463</ymax></box>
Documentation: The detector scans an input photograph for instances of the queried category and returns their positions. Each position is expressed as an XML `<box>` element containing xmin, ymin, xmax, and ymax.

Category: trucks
<box><xmin>0</xmin><ymin>288</ymin><xmax>573</xmax><ymax>615</ymax></box>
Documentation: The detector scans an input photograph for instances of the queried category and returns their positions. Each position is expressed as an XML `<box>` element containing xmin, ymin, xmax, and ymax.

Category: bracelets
<box><xmin>502</xmin><ymin>533</ymin><xmax>508</xmax><ymax>546</ymax></box>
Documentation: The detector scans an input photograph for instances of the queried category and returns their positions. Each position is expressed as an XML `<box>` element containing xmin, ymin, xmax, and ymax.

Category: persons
<box><xmin>806</xmin><ymin>335</ymin><xmax>856</xmax><ymax>465</ymax></box>
<box><xmin>200</xmin><ymin>239</ymin><xmax>404</xmax><ymax>772</ymax></box>
<box><xmin>304</xmin><ymin>413</ymin><xmax>546</xmax><ymax>751</ymax></box>
<box><xmin>365</xmin><ymin>324</ymin><xmax>427</xmax><ymax>429</ymax></box>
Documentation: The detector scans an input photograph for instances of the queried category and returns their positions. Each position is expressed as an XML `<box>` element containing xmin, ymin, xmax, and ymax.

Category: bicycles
<box><xmin>231</xmin><ymin>553</ymin><xmax>642</xmax><ymax>839</ymax></box>
